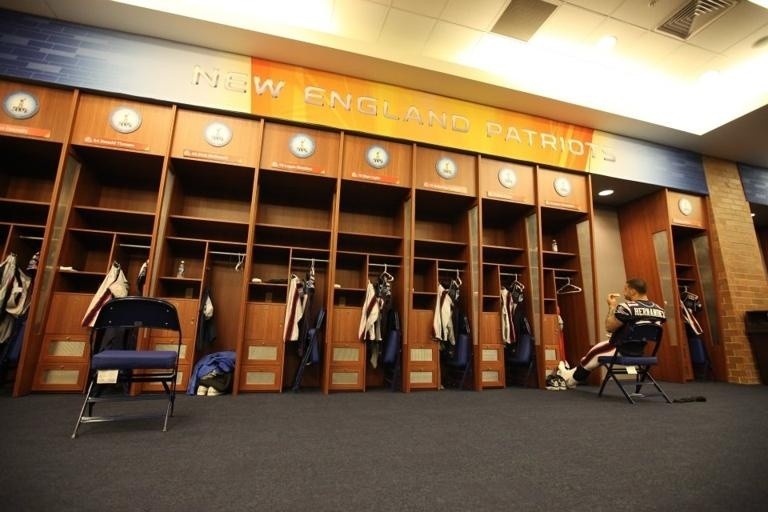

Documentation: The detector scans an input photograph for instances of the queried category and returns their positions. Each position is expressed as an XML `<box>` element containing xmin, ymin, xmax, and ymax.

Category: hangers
<box><xmin>679</xmin><ymin>282</ymin><xmax>701</xmax><ymax>301</ymax></box>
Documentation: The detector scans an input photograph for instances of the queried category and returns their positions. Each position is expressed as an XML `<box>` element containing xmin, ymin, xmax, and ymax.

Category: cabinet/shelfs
<box><xmin>1</xmin><ymin>131</ymin><xmax>602</xmax><ymax>401</ymax></box>
<box><xmin>671</xmin><ymin>235</ymin><xmax>721</xmax><ymax>384</ymax></box>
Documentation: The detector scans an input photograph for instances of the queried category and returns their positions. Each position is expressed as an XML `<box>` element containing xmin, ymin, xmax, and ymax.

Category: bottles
<box><xmin>552</xmin><ymin>240</ymin><xmax>558</xmax><ymax>252</ymax></box>
<box><xmin>177</xmin><ymin>261</ymin><xmax>185</xmax><ymax>278</ymax></box>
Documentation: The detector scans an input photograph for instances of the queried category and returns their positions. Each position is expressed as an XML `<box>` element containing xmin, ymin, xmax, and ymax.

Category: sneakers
<box><xmin>544</xmin><ymin>374</ymin><xmax>567</xmax><ymax>391</ymax></box>
<box><xmin>196</xmin><ymin>385</ymin><xmax>222</xmax><ymax>396</ymax></box>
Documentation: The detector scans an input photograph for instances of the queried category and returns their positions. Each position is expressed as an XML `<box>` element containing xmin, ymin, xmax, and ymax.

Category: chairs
<box><xmin>598</xmin><ymin>311</ymin><xmax>674</xmax><ymax>406</ymax></box>
<box><xmin>70</xmin><ymin>292</ymin><xmax>183</xmax><ymax>443</ymax></box>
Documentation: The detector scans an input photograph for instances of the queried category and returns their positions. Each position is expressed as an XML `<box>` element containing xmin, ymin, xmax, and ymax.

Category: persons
<box><xmin>558</xmin><ymin>279</ymin><xmax>667</xmax><ymax>389</ymax></box>
